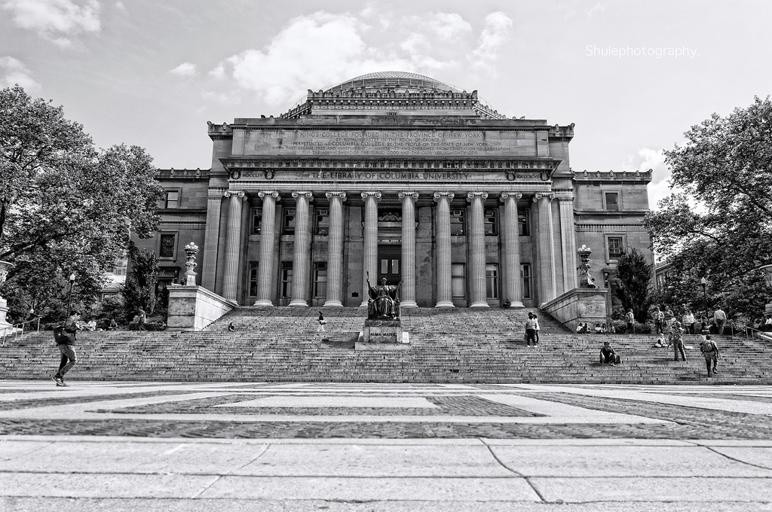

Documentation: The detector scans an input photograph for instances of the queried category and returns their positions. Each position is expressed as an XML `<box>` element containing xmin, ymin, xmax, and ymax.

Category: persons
<box><xmin>228</xmin><ymin>322</ymin><xmax>235</xmax><ymax>332</ymax></box>
<box><xmin>594</xmin><ymin>322</ymin><xmax>616</xmax><ymax>334</ymax></box>
<box><xmin>138</xmin><ymin>305</ymin><xmax>145</xmax><ymax>331</ymax></box>
<box><xmin>51</xmin><ymin>310</ymin><xmax>81</xmax><ymax>386</ymax></box>
<box><xmin>575</xmin><ymin>322</ymin><xmax>591</xmax><ymax>334</ymax></box>
<box><xmin>650</xmin><ymin>303</ymin><xmax>727</xmax><ymax>335</ymax></box>
<box><xmin>624</xmin><ymin>308</ymin><xmax>635</xmax><ymax>334</ymax></box>
<box><xmin>108</xmin><ymin>319</ymin><xmax>118</xmax><ymax>330</ymax></box>
<box><xmin>367</xmin><ymin>274</ymin><xmax>404</xmax><ymax>320</ymax></box>
<box><xmin>700</xmin><ymin>335</ymin><xmax>719</xmax><ymax>377</ymax></box>
<box><xmin>667</xmin><ymin>321</ymin><xmax>687</xmax><ymax>361</ymax></box>
<box><xmin>316</xmin><ymin>310</ymin><xmax>326</xmax><ymax>332</ymax></box>
<box><xmin>75</xmin><ymin>319</ymin><xmax>97</xmax><ymax>332</ymax></box>
<box><xmin>502</xmin><ymin>296</ymin><xmax>511</xmax><ymax>309</ymax></box>
<box><xmin>600</xmin><ymin>341</ymin><xmax>617</xmax><ymax>367</ymax></box>
<box><xmin>525</xmin><ymin>312</ymin><xmax>541</xmax><ymax>347</ymax></box>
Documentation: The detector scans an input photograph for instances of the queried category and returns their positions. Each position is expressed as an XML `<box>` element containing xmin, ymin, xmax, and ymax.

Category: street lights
<box><xmin>700</xmin><ymin>276</ymin><xmax>708</xmax><ymax>318</ymax></box>
<box><xmin>67</xmin><ymin>273</ymin><xmax>76</xmax><ymax>318</ymax></box>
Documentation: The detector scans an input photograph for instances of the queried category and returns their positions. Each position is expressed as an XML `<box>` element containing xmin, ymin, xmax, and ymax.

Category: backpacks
<box><xmin>53</xmin><ymin>323</ymin><xmax>65</xmax><ymax>343</ymax></box>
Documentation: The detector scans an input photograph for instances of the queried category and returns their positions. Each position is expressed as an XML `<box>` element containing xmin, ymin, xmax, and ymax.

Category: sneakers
<box><xmin>53</xmin><ymin>374</ymin><xmax>67</xmax><ymax>386</ymax></box>
